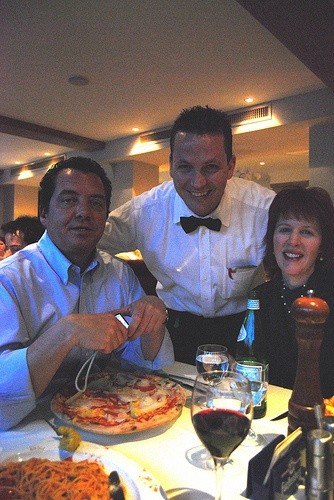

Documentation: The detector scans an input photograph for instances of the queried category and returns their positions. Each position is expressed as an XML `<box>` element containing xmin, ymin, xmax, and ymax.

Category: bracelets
<box><xmin>162</xmin><ymin>310</ymin><xmax>169</xmax><ymax>325</ymax></box>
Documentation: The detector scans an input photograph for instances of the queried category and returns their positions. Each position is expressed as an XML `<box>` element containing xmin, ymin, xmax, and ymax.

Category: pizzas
<box><xmin>51</xmin><ymin>371</ymin><xmax>186</xmax><ymax>435</ymax></box>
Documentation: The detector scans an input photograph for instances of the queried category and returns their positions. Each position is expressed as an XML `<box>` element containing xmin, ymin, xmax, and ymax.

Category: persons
<box><xmin>243</xmin><ymin>186</ymin><xmax>334</xmax><ymax>396</ymax></box>
<box><xmin>0</xmin><ymin>215</ymin><xmax>46</xmax><ymax>261</ymax></box>
<box><xmin>96</xmin><ymin>104</ymin><xmax>278</xmax><ymax>366</ymax></box>
<box><xmin>0</xmin><ymin>156</ymin><xmax>175</xmax><ymax>433</ymax></box>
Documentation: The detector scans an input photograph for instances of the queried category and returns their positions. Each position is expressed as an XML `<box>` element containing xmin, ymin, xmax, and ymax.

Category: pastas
<box><xmin>0</xmin><ymin>458</ymin><xmax>125</xmax><ymax>500</ymax></box>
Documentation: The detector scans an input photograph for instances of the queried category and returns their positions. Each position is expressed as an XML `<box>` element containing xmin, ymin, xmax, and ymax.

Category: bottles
<box><xmin>322</xmin><ymin>416</ymin><xmax>334</xmax><ymax>439</ymax></box>
<box><xmin>236</xmin><ymin>291</ymin><xmax>267</xmax><ymax>419</ymax></box>
<box><xmin>306</xmin><ymin>430</ymin><xmax>333</xmax><ymax>500</ymax></box>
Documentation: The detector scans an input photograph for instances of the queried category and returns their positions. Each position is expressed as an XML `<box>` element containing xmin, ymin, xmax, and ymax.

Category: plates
<box><xmin>0</xmin><ymin>439</ymin><xmax>169</xmax><ymax>500</ymax></box>
<box><xmin>50</xmin><ymin>397</ymin><xmax>70</xmax><ymax>423</ymax></box>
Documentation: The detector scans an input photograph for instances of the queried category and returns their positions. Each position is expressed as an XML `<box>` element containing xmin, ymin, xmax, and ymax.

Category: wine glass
<box><xmin>195</xmin><ymin>345</ymin><xmax>229</xmax><ymax>407</ymax></box>
<box><xmin>190</xmin><ymin>370</ymin><xmax>253</xmax><ymax>500</ymax></box>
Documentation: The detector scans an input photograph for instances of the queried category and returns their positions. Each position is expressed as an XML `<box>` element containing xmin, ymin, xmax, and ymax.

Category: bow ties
<box><xmin>180</xmin><ymin>216</ymin><xmax>221</xmax><ymax>234</ymax></box>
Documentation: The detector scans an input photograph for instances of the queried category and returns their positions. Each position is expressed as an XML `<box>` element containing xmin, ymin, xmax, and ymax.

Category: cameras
<box><xmin>114</xmin><ymin>313</ymin><xmax>130</xmax><ymax>329</ymax></box>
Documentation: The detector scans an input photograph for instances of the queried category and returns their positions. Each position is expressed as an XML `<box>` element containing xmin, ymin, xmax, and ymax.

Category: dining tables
<box><xmin>0</xmin><ymin>361</ymin><xmax>324</xmax><ymax>500</ymax></box>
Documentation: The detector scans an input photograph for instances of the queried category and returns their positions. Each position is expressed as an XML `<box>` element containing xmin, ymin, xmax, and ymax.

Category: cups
<box><xmin>232</xmin><ymin>361</ymin><xmax>267</xmax><ymax>419</ymax></box>
